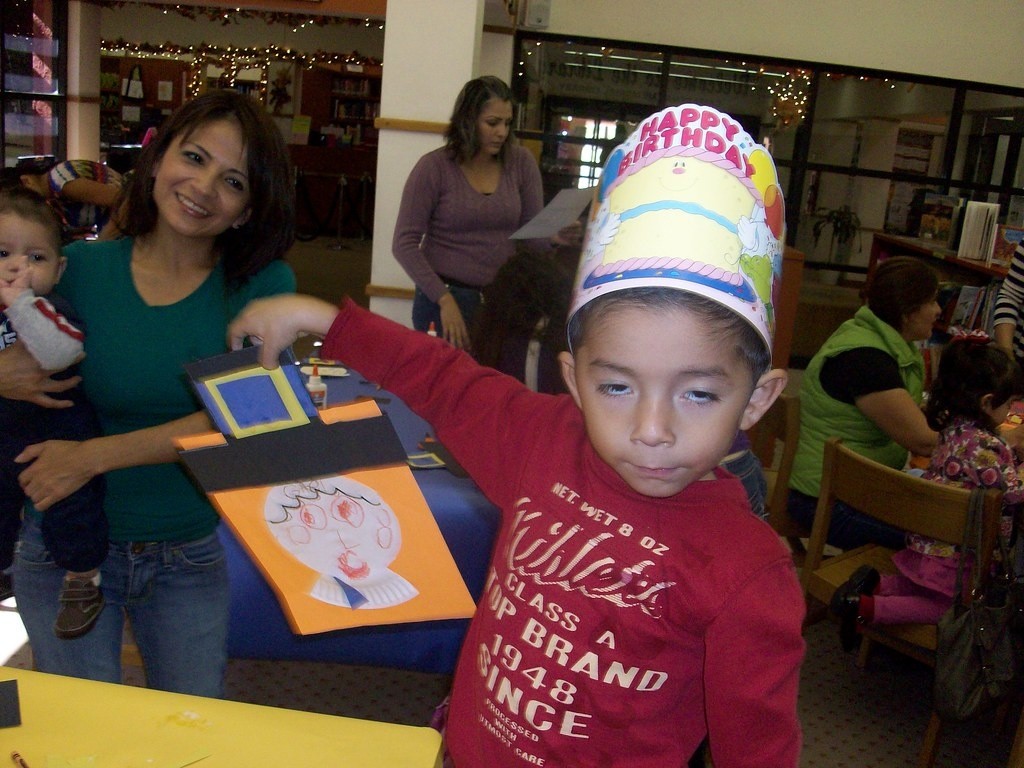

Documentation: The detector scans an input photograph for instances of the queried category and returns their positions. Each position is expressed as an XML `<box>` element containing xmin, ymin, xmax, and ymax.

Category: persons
<box><xmin>228</xmin><ymin>279</ymin><xmax>806</xmax><ymax>768</ymax></box>
<box><xmin>794</xmin><ymin>254</ymin><xmax>941</xmax><ymax>553</ymax></box>
<box><xmin>391</xmin><ymin>74</ymin><xmax>577</xmax><ymax>393</ymax></box>
<box><xmin>716</xmin><ymin>430</ymin><xmax>772</xmax><ymax>521</ymax></box>
<box><xmin>0</xmin><ymin>180</ymin><xmax>112</xmax><ymax>639</ymax></box>
<box><xmin>827</xmin><ymin>335</ymin><xmax>1024</xmax><ymax>649</ymax></box>
<box><xmin>994</xmin><ymin>235</ymin><xmax>1024</xmax><ymax>392</ymax></box>
<box><xmin>0</xmin><ymin>160</ymin><xmax>130</xmax><ymax>236</ymax></box>
<box><xmin>0</xmin><ymin>87</ymin><xmax>300</xmax><ymax>690</ymax></box>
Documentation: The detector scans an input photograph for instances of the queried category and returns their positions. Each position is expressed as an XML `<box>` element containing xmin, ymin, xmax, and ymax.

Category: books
<box><xmin>918</xmin><ymin>193</ymin><xmax>1024</xmax><ymax>270</ymax></box>
<box><xmin>948</xmin><ymin>282</ymin><xmax>999</xmax><ymax>335</ymax></box>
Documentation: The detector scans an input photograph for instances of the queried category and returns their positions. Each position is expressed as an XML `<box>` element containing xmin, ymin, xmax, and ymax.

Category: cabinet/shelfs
<box><xmin>771</xmin><ymin>245</ymin><xmax>804</xmax><ymax>372</ymax></box>
<box><xmin>99</xmin><ymin>88</ymin><xmax>120</xmax><ymax>113</ymax></box>
<box><xmin>331</xmin><ymin>92</ymin><xmax>380</xmax><ymax>126</ymax></box>
<box><xmin>859</xmin><ymin>232</ymin><xmax>1009</xmax><ymax>343</ymax></box>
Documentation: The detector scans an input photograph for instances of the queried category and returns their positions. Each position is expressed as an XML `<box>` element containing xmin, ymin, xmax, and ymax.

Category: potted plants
<box><xmin>810</xmin><ymin>205</ymin><xmax>864</xmax><ymax>274</ymax></box>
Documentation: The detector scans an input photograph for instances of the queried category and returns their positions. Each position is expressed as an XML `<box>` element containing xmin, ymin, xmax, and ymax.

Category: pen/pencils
<box><xmin>11</xmin><ymin>751</ymin><xmax>29</xmax><ymax>768</ymax></box>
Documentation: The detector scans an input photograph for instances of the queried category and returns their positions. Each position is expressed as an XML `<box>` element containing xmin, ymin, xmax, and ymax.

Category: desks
<box><xmin>0</xmin><ymin>665</ymin><xmax>445</xmax><ymax>768</ymax></box>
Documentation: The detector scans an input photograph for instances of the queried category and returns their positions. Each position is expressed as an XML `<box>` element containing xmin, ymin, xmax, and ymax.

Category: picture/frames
<box><xmin>267</xmin><ymin>62</ymin><xmax>301</xmax><ymax>116</ymax></box>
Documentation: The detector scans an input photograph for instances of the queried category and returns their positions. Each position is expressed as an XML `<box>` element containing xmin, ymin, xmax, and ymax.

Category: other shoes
<box><xmin>840</xmin><ymin>596</ymin><xmax>863</xmax><ymax>655</ymax></box>
<box><xmin>54</xmin><ymin>577</ymin><xmax>104</xmax><ymax>640</ymax></box>
<box><xmin>831</xmin><ymin>566</ymin><xmax>880</xmax><ymax>614</ymax></box>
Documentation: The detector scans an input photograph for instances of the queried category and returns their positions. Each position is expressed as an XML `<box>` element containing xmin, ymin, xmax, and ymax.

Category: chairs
<box><xmin>800</xmin><ymin>435</ymin><xmax>1024</xmax><ymax>768</ymax></box>
<box><xmin>752</xmin><ymin>391</ymin><xmax>809</xmax><ymax>538</ymax></box>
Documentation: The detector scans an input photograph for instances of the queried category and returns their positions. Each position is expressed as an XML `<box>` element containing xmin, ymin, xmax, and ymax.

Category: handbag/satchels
<box><xmin>932</xmin><ymin>493</ymin><xmax>1015</xmax><ymax>720</ymax></box>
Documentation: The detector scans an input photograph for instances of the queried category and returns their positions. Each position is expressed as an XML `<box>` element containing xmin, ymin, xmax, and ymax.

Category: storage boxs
<box><xmin>994</xmin><ymin>423</ymin><xmax>1019</xmax><ymax>449</ymax></box>
